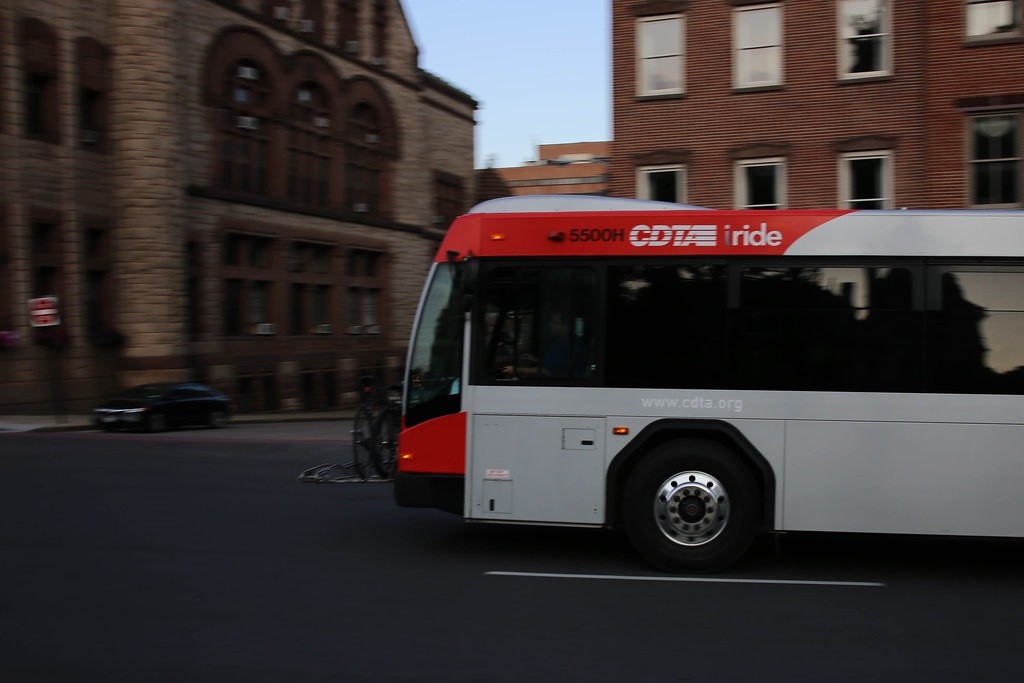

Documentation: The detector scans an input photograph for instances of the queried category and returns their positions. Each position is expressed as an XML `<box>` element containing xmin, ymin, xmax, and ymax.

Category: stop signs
<box><xmin>25</xmin><ymin>297</ymin><xmax>62</xmax><ymax>328</ymax></box>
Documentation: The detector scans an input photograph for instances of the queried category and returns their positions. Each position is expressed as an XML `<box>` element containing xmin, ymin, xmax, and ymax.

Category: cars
<box><xmin>89</xmin><ymin>382</ymin><xmax>234</xmax><ymax>433</ymax></box>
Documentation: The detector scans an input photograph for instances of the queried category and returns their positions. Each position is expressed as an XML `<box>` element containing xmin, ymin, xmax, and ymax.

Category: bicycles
<box><xmin>351</xmin><ymin>374</ymin><xmax>400</xmax><ymax>481</ymax></box>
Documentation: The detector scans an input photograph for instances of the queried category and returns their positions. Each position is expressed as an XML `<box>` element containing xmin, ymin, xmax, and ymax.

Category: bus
<box><xmin>297</xmin><ymin>195</ymin><xmax>1024</xmax><ymax>581</ymax></box>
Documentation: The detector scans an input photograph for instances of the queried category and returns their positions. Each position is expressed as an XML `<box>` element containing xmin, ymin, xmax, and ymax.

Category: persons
<box><xmin>502</xmin><ymin>315</ymin><xmax>588</xmax><ymax>376</ymax></box>
